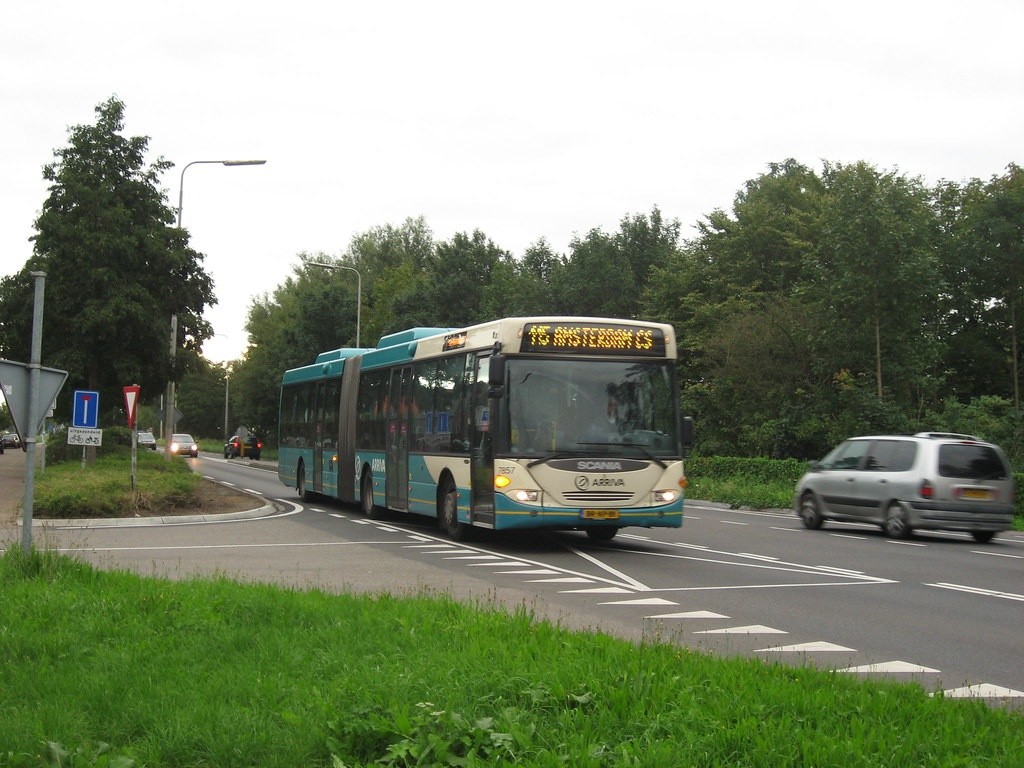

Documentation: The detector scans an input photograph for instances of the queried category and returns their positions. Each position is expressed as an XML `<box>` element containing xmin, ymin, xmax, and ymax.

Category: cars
<box><xmin>224</xmin><ymin>432</ymin><xmax>262</xmax><ymax>460</ymax></box>
<box><xmin>171</xmin><ymin>433</ymin><xmax>199</xmax><ymax>458</ymax></box>
<box><xmin>137</xmin><ymin>430</ymin><xmax>156</xmax><ymax>450</ymax></box>
<box><xmin>0</xmin><ymin>432</ymin><xmax>21</xmax><ymax>455</ymax></box>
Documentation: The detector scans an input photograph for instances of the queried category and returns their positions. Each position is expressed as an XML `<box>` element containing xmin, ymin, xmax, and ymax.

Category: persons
<box><xmin>590</xmin><ymin>397</ymin><xmax>633</xmax><ymax>433</ymax></box>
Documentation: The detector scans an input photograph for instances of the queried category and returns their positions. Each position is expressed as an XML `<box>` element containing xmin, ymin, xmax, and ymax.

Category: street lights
<box><xmin>166</xmin><ymin>159</ymin><xmax>265</xmax><ymax>464</ymax></box>
<box><xmin>306</xmin><ymin>261</ymin><xmax>361</xmax><ymax>348</ymax></box>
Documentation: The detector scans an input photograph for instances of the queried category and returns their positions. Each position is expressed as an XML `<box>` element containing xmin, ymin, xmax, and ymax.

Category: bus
<box><xmin>278</xmin><ymin>313</ymin><xmax>687</xmax><ymax>546</ymax></box>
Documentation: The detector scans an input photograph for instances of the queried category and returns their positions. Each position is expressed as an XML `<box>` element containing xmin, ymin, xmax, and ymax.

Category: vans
<box><xmin>795</xmin><ymin>431</ymin><xmax>1018</xmax><ymax>540</ymax></box>
